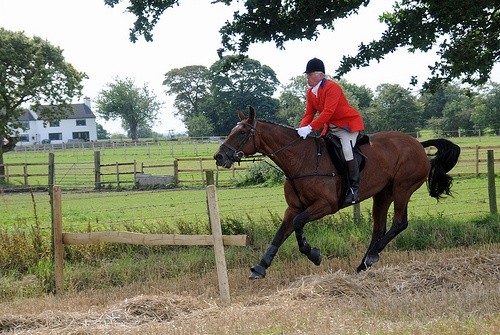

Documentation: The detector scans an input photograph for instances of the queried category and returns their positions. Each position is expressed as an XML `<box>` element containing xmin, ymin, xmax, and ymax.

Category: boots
<box><xmin>344</xmin><ymin>158</ymin><xmax>361</xmax><ymax>204</ymax></box>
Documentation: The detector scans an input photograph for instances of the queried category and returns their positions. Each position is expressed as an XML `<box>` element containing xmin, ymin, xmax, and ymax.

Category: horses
<box><xmin>212</xmin><ymin>104</ymin><xmax>462</xmax><ymax>281</ymax></box>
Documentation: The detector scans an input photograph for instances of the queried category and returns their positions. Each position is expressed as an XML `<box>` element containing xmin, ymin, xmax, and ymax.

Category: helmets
<box><xmin>304</xmin><ymin>58</ymin><xmax>325</xmax><ymax>74</ymax></box>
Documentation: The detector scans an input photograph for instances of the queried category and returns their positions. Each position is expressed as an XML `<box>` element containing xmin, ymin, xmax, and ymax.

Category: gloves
<box><xmin>296</xmin><ymin>124</ymin><xmax>312</xmax><ymax>140</ymax></box>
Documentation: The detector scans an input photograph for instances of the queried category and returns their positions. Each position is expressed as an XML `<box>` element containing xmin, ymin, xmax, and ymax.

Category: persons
<box><xmin>295</xmin><ymin>57</ymin><xmax>364</xmax><ymax>206</ymax></box>
